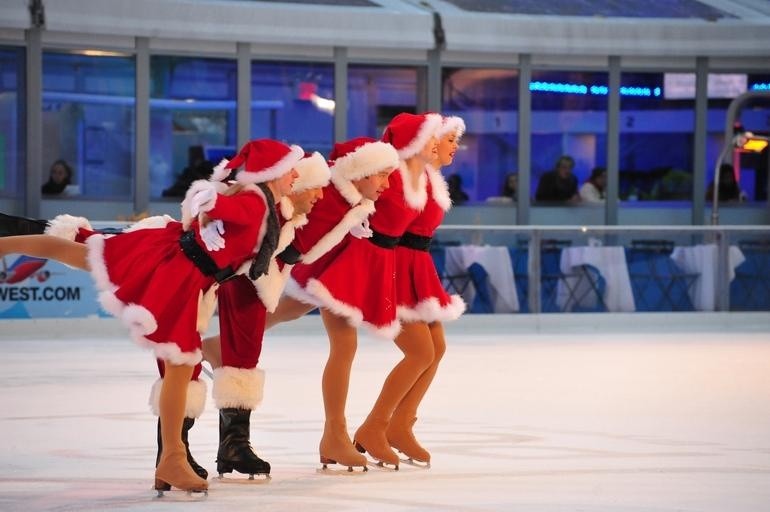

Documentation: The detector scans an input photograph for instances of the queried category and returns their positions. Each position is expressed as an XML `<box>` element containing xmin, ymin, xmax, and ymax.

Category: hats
<box><xmin>420</xmin><ymin>111</ymin><xmax>466</xmax><ymax>138</ymax></box>
<box><xmin>328</xmin><ymin>136</ymin><xmax>398</xmax><ymax>181</ymax></box>
<box><xmin>209</xmin><ymin>139</ymin><xmax>303</xmax><ymax>187</ymax></box>
<box><xmin>285</xmin><ymin>139</ymin><xmax>331</xmax><ymax>192</ymax></box>
<box><xmin>378</xmin><ymin>111</ymin><xmax>441</xmax><ymax>158</ymax></box>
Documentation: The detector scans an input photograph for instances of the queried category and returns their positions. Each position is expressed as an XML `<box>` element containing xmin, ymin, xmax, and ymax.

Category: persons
<box><xmin>0</xmin><ymin>112</ymin><xmax>468</xmax><ymax>496</ymax></box>
<box><xmin>578</xmin><ymin>167</ymin><xmax>608</xmax><ymax>204</ymax></box>
<box><xmin>536</xmin><ymin>155</ymin><xmax>579</xmax><ymax>202</ymax></box>
<box><xmin>504</xmin><ymin>173</ymin><xmax>518</xmax><ymax>199</ymax></box>
<box><xmin>707</xmin><ymin>164</ymin><xmax>739</xmax><ymax>204</ymax></box>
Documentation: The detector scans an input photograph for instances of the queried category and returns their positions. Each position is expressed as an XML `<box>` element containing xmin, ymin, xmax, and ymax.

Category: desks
<box><xmin>559</xmin><ymin>244</ymin><xmax>634</xmax><ymax>308</ymax></box>
<box><xmin>444</xmin><ymin>244</ymin><xmax>520</xmax><ymax>310</ymax></box>
<box><xmin>672</xmin><ymin>241</ymin><xmax>744</xmax><ymax>309</ymax></box>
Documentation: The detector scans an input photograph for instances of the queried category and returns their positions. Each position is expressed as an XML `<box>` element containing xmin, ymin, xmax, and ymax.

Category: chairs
<box><xmin>729</xmin><ymin>242</ymin><xmax>768</xmax><ymax>309</ymax></box>
<box><xmin>438</xmin><ymin>253</ymin><xmax>500</xmax><ymax>309</ymax></box>
<box><xmin>631</xmin><ymin>239</ymin><xmax>700</xmax><ymax>310</ymax></box>
<box><xmin>516</xmin><ymin>261</ymin><xmax>608</xmax><ymax>310</ymax></box>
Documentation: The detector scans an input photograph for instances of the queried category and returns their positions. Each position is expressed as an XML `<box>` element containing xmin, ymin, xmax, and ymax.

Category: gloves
<box><xmin>189</xmin><ymin>187</ymin><xmax>218</xmax><ymax>219</ymax></box>
<box><xmin>349</xmin><ymin>219</ymin><xmax>375</xmax><ymax>241</ymax></box>
<box><xmin>196</xmin><ymin>210</ymin><xmax>228</xmax><ymax>253</ymax></box>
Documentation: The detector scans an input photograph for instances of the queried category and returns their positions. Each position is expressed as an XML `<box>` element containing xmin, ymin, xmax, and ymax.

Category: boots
<box><xmin>387</xmin><ymin>415</ymin><xmax>430</xmax><ymax>462</ymax></box>
<box><xmin>150</xmin><ymin>379</ymin><xmax>207</xmax><ymax>489</ymax></box>
<box><xmin>1</xmin><ymin>207</ymin><xmax>93</xmax><ymax>266</ymax></box>
<box><xmin>211</xmin><ymin>361</ymin><xmax>271</xmax><ymax>475</ymax></box>
<box><xmin>353</xmin><ymin>415</ymin><xmax>399</xmax><ymax>465</ymax></box>
<box><xmin>319</xmin><ymin>422</ymin><xmax>367</xmax><ymax>465</ymax></box>
<box><xmin>155</xmin><ymin>448</ymin><xmax>209</xmax><ymax>490</ymax></box>
